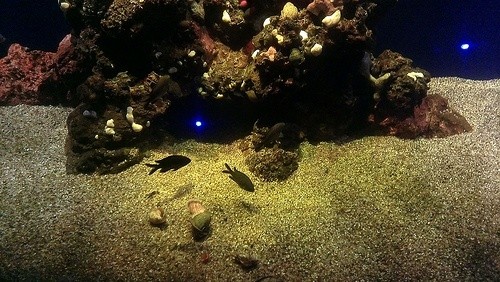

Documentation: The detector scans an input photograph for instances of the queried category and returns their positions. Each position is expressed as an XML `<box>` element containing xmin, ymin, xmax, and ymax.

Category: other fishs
<box><xmin>145</xmin><ymin>154</ymin><xmax>192</xmax><ymax>176</ymax></box>
<box><xmin>221</xmin><ymin>162</ymin><xmax>256</xmax><ymax>193</ymax></box>
<box><xmin>167</xmin><ymin>182</ymin><xmax>195</xmax><ymax>202</ymax></box>
<box><xmin>253</xmin><ymin>121</ymin><xmax>286</xmax><ymax>152</ymax></box>
